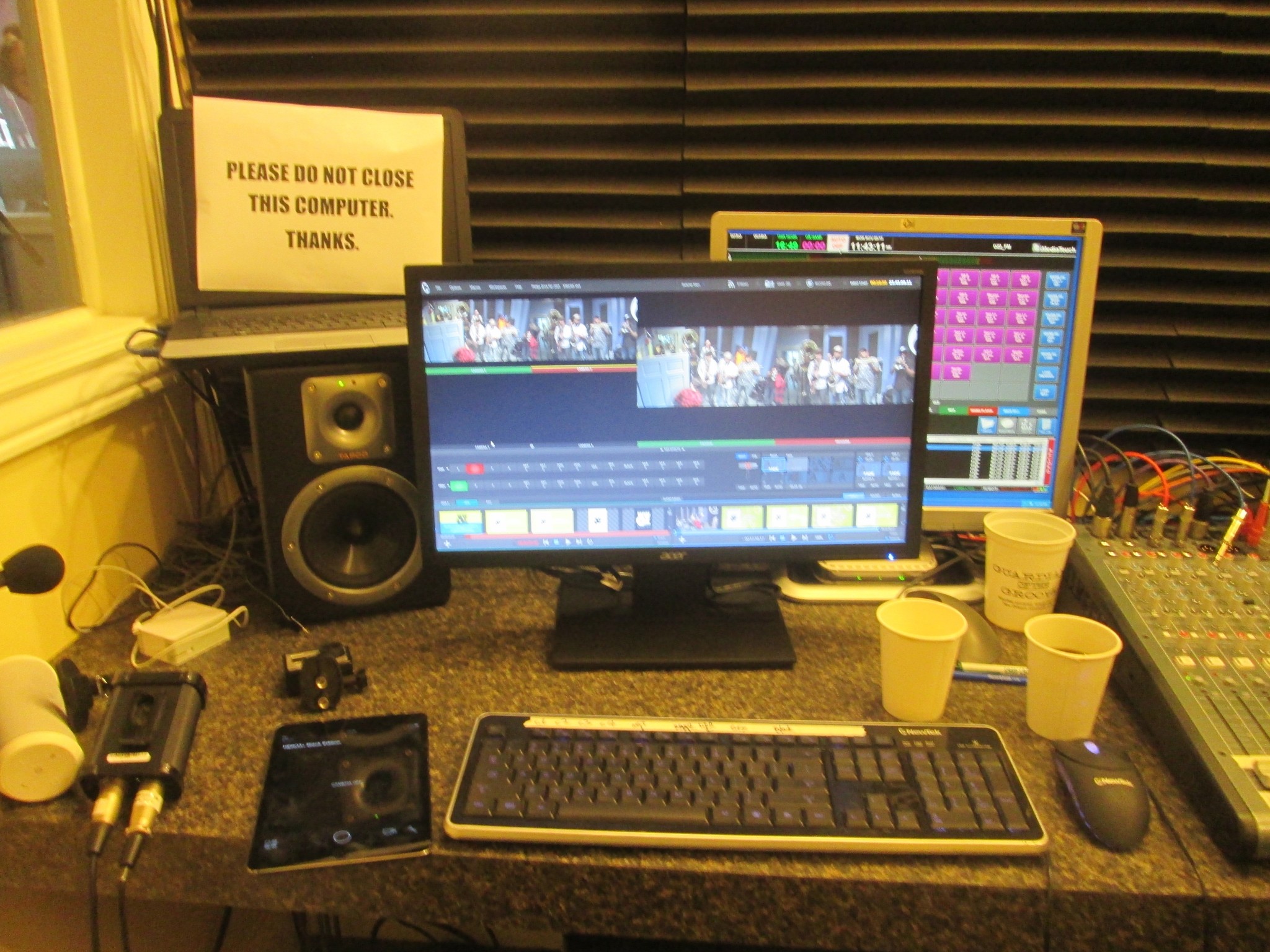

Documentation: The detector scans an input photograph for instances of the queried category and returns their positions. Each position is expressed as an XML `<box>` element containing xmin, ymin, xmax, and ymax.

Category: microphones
<box><xmin>1</xmin><ymin>543</ymin><xmax>67</xmax><ymax>596</ymax></box>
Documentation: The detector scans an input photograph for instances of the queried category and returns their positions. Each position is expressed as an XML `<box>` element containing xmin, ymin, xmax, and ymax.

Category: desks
<box><xmin>2</xmin><ymin>527</ymin><xmax>1268</xmax><ymax>952</ymax></box>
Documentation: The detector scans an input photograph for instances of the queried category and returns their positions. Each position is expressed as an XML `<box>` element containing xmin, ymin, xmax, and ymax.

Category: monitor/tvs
<box><xmin>398</xmin><ymin>258</ymin><xmax>940</xmax><ymax>564</ymax></box>
<box><xmin>707</xmin><ymin>206</ymin><xmax>1104</xmax><ymax>536</ymax></box>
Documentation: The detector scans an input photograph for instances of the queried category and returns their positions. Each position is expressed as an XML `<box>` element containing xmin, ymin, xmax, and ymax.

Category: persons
<box><xmin>435</xmin><ymin>304</ymin><xmax>635</xmax><ymax>363</ymax></box>
<box><xmin>890</xmin><ymin>346</ymin><xmax>915</xmax><ymax>404</ymax></box>
<box><xmin>642</xmin><ymin>327</ymin><xmax>882</xmax><ymax>407</ymax></box>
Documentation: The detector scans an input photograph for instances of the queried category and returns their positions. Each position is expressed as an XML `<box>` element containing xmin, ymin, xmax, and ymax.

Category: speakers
<box><xmin>229</xmin><ymin>349</ymin><xmax>452</xmax><ymax>630</ymax></box>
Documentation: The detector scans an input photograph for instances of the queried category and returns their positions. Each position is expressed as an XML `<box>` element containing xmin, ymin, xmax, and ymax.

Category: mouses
<box><xmin>1053</xmin><ymin>735</ymin><xmax>1154</xmax><ymax>856</ymax></box>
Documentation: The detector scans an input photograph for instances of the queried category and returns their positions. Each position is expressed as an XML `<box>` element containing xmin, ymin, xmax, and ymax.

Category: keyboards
<box><xmin>437</xmin><ymin>711</ymin><xmax>1053</xmax><ymax>861</ymax></box>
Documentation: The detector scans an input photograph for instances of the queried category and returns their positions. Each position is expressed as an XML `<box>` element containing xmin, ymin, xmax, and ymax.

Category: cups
<box><xmin>875</xmin><ymin>597</ymin><xmax>968</xmax><ymax>722</ymax></box>
<box><xmin>983</xmin><ymin>507</ymin><xmax>1077</xmax><ymax>636</ymax></box>
<box><xmin>1021</xmin><ymin>614</ymin><xmax>1125</xmax><ymax>738</ymax></box>
<box><xmin>1</xmin><ymin>654</ymin><xmax>85</xmax><ymax>802</ymax></box>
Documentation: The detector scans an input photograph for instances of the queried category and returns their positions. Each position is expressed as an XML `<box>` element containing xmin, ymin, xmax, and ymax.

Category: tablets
<box><xmin>246</xmin><ymin>711</ymin><xmax>432</xmax><ymax>876</ymax></box>
